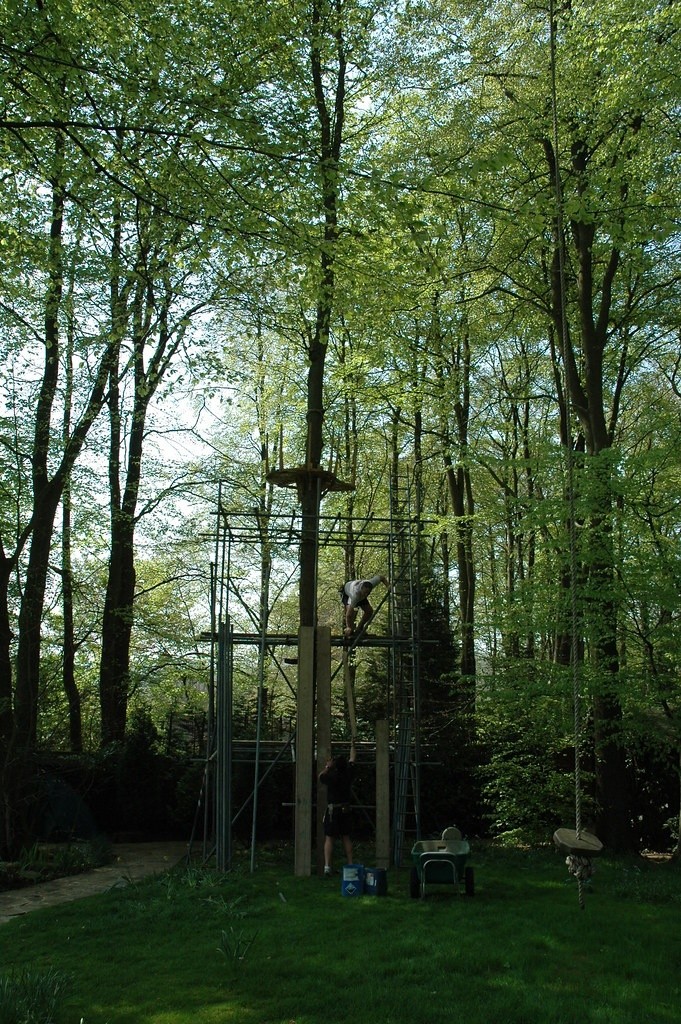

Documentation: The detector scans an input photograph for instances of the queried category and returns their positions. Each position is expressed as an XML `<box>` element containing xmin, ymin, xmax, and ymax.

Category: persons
<box><xmin>342</xmin><ymin>575</ymin><xmax>392</xmax><ymax>634</ymax></box>
<box><xmin>318</xmin><ymin>735</ymin><xmax>357</xmax><ymax>876</ymax></box>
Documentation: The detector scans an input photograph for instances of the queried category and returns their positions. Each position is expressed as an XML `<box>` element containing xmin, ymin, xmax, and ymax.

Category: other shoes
<box><xmin>355</xmin><ymin>629</ymin><xmax>368</xmax><ymax>636</ymax></box>
<box><xmin>324</xmin><ymin>866</ymin><xmax>333</xmax><ymax>874</ymax></box>
